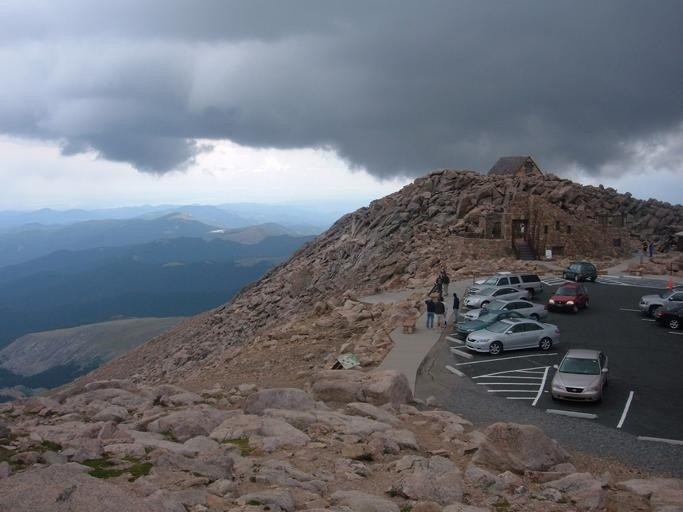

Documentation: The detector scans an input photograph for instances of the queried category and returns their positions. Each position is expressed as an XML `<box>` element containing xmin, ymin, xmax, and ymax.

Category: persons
<box><xmin>425</xmin><ymin>299</ymin><xmax>434</xmax><ymax>329</ymax></box>
<box><xmin>520</xmin><ymin>224</ymin><xmax>527</xmax><ymax>243</ymax></box>
<box><xmin>435</xmin><ymin>298</ymin><xmax>447</xmax><ymax>328</ymax></box>
<box><xmin>436</xmin><ymin>267</ymin><xmax>448</xmax><ymax>298</ymax></box>
<box><xmin>452</xmin><ymin>293</ymin><xmax>460</xmax><ymax>324</ymax></box>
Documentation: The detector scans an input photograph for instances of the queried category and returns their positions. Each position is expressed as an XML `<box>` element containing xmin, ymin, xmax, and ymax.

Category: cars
<box><xmin>638</xmin><ymin>288</ymin><xmax>683</xmax><ymax>329</ymax></box>
<box><xmin>455</xmin><ymin>263</ymin><xmax>608</xmax><ymax>404</ymax></box>
